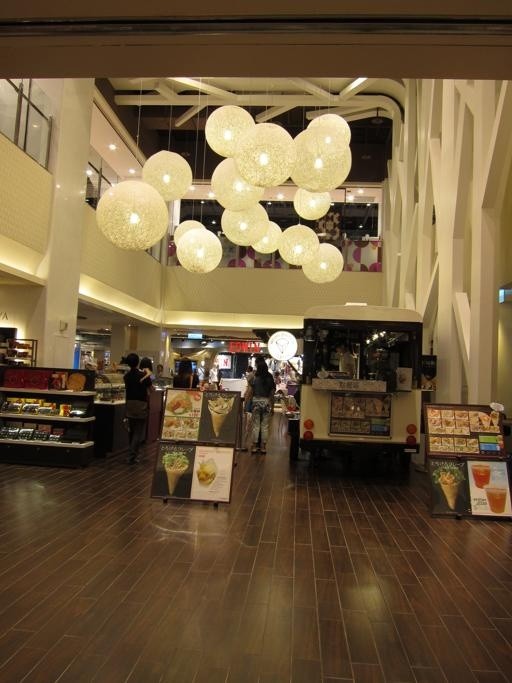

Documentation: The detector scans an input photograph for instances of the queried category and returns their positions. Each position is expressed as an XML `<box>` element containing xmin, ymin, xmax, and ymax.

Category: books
<box><xmin>427</xmin><ymin>407</ymin><xmax>481</xmax><ymax>453</ymax></box>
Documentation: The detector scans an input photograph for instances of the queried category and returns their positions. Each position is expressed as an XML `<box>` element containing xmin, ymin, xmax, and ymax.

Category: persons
<box><xmin>157</xmin><ymin>363</ymin><xmax>163</xmax><ymax>375</ymax></box>
<box><xmin>334</xmin><ymin>336</ymin><xmax>357</xmax><ymax>379</ymax></box>
<box><xmin>243</xmin><ymin>356</ymin><xmax>275</xmax><ymax>453</ymax></box>
<box><xmin>209</xmin><ymin>362</ymin><xmax>218</xmax><ymax>384</ymax></box>
<box><xmin>173</xmin><ymin>357</ymin><xmax>199</xmax><ymax>387</ymax></box>
<box><xmin>123</xmin><ymin>353</ymin><xmax>155</xmax><ymax>465</ymax></box>
<box><xmin>198</xmin><ymin>360</ymin><xmax>207</xmax><ymax>380</ymax></box>
<box><xmin>140</xmin><ymin>356</ymin><xmax>155</xmax><ymax>409</ymax></box>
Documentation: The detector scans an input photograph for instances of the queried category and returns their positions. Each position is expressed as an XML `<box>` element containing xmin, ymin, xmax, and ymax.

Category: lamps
<box><xmin>266</xmin><ymin>329</ymin><xmax>298</xmax><ymax>361</ymax></box>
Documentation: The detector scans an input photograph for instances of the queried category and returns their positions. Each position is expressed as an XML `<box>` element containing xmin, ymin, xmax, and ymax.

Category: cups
<box><xmin>482</xmin><ymin>483</ymin><xmax>507</xmax><ymax>514</ymax></box>
<box><xmin>471</xmin><ymin>463</ymin><xmax>491</xmax><ymax>488</ymax></box>
<box><xmin>368</xmin><ymin>372</ymin><xmax>377</xmax><ymax>380</ymax></box>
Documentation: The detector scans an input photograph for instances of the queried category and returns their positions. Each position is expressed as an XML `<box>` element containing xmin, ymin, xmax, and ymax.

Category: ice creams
<box><xmin>165</xmin><ymin>452</ymin><xmax>188</xmax><ymax>494</ymax></box>
<box><xmin>208</xmin><ymin>398</ymin><xmax>234</xmax><ymax>436</ymax></box>
<box><xmin>439</xmin><ymin>472</ymin><xmax>460</xmax><ymax>509</ymax></box>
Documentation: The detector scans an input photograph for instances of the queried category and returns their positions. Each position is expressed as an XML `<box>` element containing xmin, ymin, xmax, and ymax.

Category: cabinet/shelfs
<box><xmin>0</xmin><ymin>326</ymin><xmax>169</xmax><ymax>470</ymax></box>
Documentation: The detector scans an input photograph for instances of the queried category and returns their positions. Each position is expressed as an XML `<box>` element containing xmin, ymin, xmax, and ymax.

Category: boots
<box><xmin>126</xmin><ymin>447</ymin><xmax>134</xmax><ymax>461</ymax></box>
<box><xmin>128</xmin><ymin>452</ymin><xmax>138</xmax><ymax>465</ymax></box>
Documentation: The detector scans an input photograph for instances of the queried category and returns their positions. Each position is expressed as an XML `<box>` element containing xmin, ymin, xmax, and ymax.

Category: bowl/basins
<box><xmin>328</xmin><ymin>370</ymin><xmax>345</xmax><ymax>379</ymax></box>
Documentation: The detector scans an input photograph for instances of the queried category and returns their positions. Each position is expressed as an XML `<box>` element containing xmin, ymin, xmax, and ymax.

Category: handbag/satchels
<box><xmin>119</xmin><ymin>416</ymin><xmax>131</xmax><ymax>433</ymax></box>
<box><xmin>246</xmin><ymin>399</ymin><xmax>253</xmax><ymax>413</ymax></box>
